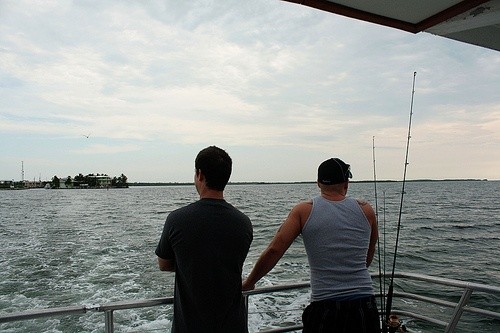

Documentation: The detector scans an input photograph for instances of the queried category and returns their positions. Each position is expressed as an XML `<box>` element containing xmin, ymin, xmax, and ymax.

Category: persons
<box><xmin>154</xmin><ymin>147</ymin><xmax>255</xmax><ymax>333</ymax></box>
<box><xmin>240</xmin><ymin>156</ymin><xmax>381</xmax><ymax>333</ymax></box>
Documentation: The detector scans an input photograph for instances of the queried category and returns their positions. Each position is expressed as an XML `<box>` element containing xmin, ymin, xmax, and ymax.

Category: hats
<box><xmin>318</xmin><ymin>157</ymin><xmax>350</xmax><ymax>185</ymax></box>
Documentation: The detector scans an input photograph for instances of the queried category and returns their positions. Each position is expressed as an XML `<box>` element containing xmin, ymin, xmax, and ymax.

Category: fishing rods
<box><xmin>384</xmin><ymin>191</ymin><xmax>386</xmax><ymax>333</ymax></box>
<box><xmin>385</xmin><ymin>71</ymin><xmax>416</xmax><ymax>332</ymax></box>
<box><xmin>372</xmin><ymin>135</ymin><xmax>384</xmax><ymax>332</ymax></box>
